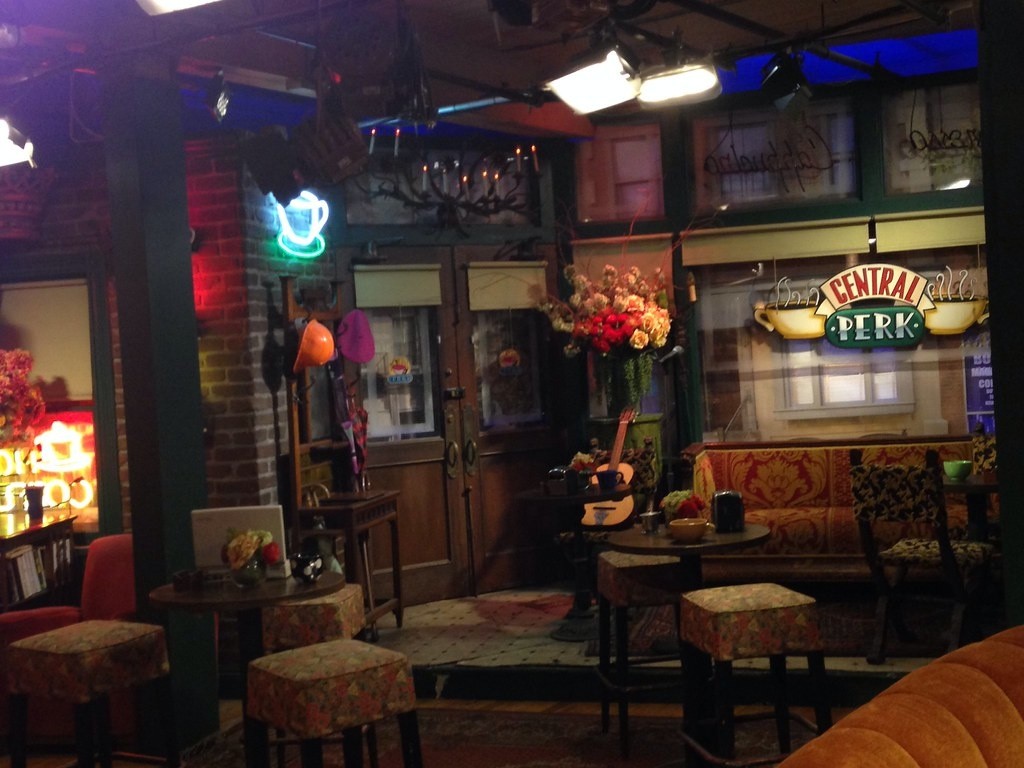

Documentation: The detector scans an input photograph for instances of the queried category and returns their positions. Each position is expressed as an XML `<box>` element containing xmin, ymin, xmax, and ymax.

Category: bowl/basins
<box><xmin>942</xmin><ymin>461</ymin><xmax>973</xmax><ymax>482</ymax></box>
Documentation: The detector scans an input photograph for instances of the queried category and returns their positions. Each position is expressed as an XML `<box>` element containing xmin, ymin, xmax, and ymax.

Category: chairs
<box><xmin>851</xmin><ymin>448</ymin><xmax>998</xmax><ymax>664</ymax></box>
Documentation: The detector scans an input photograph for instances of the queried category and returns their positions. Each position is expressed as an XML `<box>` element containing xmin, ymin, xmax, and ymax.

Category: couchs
<box><xmin>0</xmin><ymin>534</ymin><xmax>219</xmax><ymax>750</ymax></box>
<box><xmin>774</xmin><ymin>624</ymin><xmax>1024</xmax><ymax>768</ymax></box>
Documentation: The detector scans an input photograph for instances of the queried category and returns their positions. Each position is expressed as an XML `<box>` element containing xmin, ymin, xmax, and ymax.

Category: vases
<box><xmin>233</xmin><ymin>555</ymin><xmax>263</xmax><ymax>589</ymax></box>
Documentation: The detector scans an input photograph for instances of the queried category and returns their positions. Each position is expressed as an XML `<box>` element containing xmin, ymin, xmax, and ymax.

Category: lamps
<box><xmin>352</xmin><ymin>0</ymin><xmax>546</xmax><ymax>237</ymax></box>
<box><xmin>637</xmin><ymin>47</ymin><xmax>723</xmax><ymax>109</ymax></box>
<box><xmin>759</xmin><ymin>48</ymin><xmax>804</xmax><ymax>103</ymax></box>
<box><xmin>542</xmin><ymin>21</ymin><xmax>640</xmax><ymax>117</ymax></box>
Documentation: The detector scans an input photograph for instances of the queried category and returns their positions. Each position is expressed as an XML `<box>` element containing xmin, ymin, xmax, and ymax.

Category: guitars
<box><xmin>579</xmin><ymin>402</ymin><xmax>640</xmax><ymax>526</ymax></box>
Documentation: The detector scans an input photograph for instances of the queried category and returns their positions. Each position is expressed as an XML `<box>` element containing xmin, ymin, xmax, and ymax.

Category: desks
<box><xmin>146</xmin><ymin>577</ymin><xmax>326</xmax><ymax>697</ymax></box>
<box><xmin>287</xmin><ymin>491</ymin><xmax>402</xmax><ymax>639</ymax></box>
<box><xmin>599</xmin><ymin>521</ymin><xmax>772</xmax><ymax>722</ymax></box>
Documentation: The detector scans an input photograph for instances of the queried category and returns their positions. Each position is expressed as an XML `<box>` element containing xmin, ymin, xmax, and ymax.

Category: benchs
<box><xmin>680</xmin><ymin>438</ymin><xmax>997</xmax><ymax>588</ymax></box>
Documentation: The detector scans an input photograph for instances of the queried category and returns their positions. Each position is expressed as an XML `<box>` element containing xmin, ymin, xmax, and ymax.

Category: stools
<box><xmin>264</xmin><ymin>583</ymin><xmax>370</xmax><ymax>653</ymax></box>
<box><xmin>599</xmin><ymin>551</ymin><xmax>701</xmax><ymax>756</ymax></box>
<box><xmin>245</xmin><ymin>638</ymin><xmax>424</xmax><ymax>768</ymax></box>
<box><xmin>679</xmin><ymin>582</ymin><xmax>833</xmax><ymax>768</ymax></box>
<box><xmin>10</xmin><ymin>620</ymin><xmax>180</xmax><ymax>768</ymax></box>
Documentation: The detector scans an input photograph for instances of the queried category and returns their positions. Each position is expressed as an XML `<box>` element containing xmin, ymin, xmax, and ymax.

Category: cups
<box><xmin>669</xmin><ymin>518</ymin><xmax>714</xmax><ymax>542</ymax></box>
<box><xmin>288</xmin><ymin>551</ymin><xmax>324</xmax><ymax>585</ymax></box>
<box><xmin>26</xmin><ymin>486</ymin><xmax>44</xmax><ymax>520</ymax></box>
<box><xmin>639</xmin><ymin>510</ymin><xmax>662</xmax><ymax>534</ymax></box>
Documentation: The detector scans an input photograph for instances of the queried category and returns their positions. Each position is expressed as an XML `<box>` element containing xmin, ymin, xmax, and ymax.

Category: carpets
<box><xmin>172</xmin><ymin>704</ymin><xmax>814</xmax><ymax>768</ymax></box>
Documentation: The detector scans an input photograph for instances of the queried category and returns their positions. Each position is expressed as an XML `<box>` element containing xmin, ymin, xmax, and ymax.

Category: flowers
<box><xmin>0</xmin><ymin>349</ymin><xmax>46</xmax><ymax>448</ymax></box>
<box><xmin>532</xmin><ymin>266</ymin><xmax>672</xmax><ymax>406</ymax></box>
<box><xmin>222</xmin><ymin>529</ymin><xmax>282</xmax><ymax>570</ymax></box>
